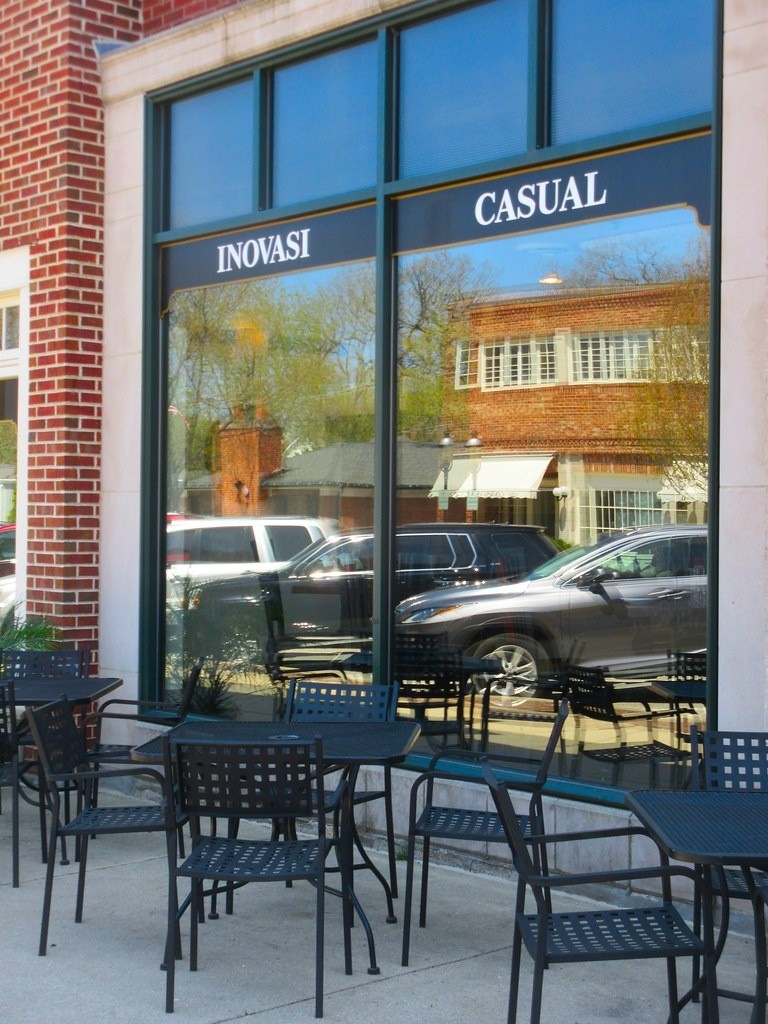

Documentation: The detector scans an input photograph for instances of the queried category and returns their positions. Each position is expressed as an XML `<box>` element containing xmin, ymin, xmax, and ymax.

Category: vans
<box><xmin>165</xmin><ymin>515</ymin><xmax>340</xmax><ymax>621</ymax></box>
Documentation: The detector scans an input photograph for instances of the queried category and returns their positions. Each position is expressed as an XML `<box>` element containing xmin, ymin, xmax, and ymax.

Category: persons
<box><xmin>650</xmin><ymin>547</ymin><xmax>679</xmax><ymax>578</ymax></box>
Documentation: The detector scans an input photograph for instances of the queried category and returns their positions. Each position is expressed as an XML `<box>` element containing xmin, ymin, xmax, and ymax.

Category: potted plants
<box><xmin>0</xmin><ymin>600</ymin><xmax>68</xmax><ymax>786</ymax></box>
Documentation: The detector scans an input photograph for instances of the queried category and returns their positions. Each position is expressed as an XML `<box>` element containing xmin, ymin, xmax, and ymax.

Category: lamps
<box><xmin>553</xmin><ymin>486</ymin><xmax>570</xmax><ymax>501</ymax></box>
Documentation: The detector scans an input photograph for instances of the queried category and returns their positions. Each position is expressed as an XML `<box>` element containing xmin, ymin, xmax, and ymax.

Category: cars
<box><xmin>0</xmin><ymin>521</ymin><xmax>16</xmax><ymax>619</ymax></box>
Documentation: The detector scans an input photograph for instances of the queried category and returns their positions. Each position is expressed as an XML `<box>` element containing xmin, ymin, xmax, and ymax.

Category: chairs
<box><xmin>690</xmin><ymin>723</ymin><xmax>768</xmax><ymax>1004</ymax></box>
<box><xmin>224</xmin><ymin>677</ymin><xmax>399</xmax><ymax>916</ymax></box>
<box><xmin>161</xmin><ymin>731</ymin><xmax>354</xmax><ymax>1018</ymax></box>
<box><xmin>480</xmin><ymin>762</ymin><xmax>721</xmax><ymax>1024</ymax></box>
<box><xmin>401</xmin><ymin>698</ymin><xmax>569</xmax><ymax>966</ymax></box>
<box><xmin>262</xmin><ymin>635</ymin><xmax>708</xmax><ymax>789</ymax></box>
<box><xmin>73</xmin><ymin>656</ymin><xmax>205</xmax><ymax>862</ymax></box>
<box><xmin>0</xmin><ymin>649</ymin><xmax>92</xmax><ymax>837</ymax></box>
<box><xmin>26</xmin><ymin>694</ymin><xmax>205</xmax><ymax>960</ymax></box>
<box><xmin>0</xmin><ymin>680</ymin><xmax>49</xmax><ymax>887</ymax></box>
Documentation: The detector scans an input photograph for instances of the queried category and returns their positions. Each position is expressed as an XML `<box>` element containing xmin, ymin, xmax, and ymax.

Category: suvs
<box><xmin>179</xmin><ymin>522</ymin><xmax>566</xmax><ymax>666</ymax></box>
<box><xmin>395</xmin><ymin>524</ymin><xmax>708</xmax><ymax>708</ymax></box>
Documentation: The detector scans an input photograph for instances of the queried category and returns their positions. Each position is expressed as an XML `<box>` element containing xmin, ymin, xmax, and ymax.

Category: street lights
<box><xmin>464</xmin><ymin>433</ymin><xmax>485</xmax><ymax>522</ymax></box>
<box><xmin>439</xmin><ymin>429</ymin><xmax>455</xmax><ymax>526</ymax></box>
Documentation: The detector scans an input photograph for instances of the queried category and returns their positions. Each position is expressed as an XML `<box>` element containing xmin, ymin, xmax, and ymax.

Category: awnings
<box><xmin>428</xmin><ymin>450</ymin><xmax>558</xmax><ymax>503</ymax></box>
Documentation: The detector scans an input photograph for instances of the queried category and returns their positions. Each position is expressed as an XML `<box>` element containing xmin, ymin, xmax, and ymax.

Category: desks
<box><xmin>646</xmin><ymin>679</ymin><xmax>707</xmax><ymax>702</ymax></box>
<box><xmin>338</xmin><ymin>646</ymin><xmax>505</xmax><ymax>756</ymax></box>
<box><xmin>625</xmin><ymin>786</ymin><xmax>768</xmax><ymax>1024</ymax></box>
<box><xmin>0</xmin><ymin>677</ymin><xmax>123</xmax><ymax>865</ymax></box>
<box><xmin>129</xmin><ymin>719</ymin><xmax>422</xmax><ymax>975</ymax></box>
